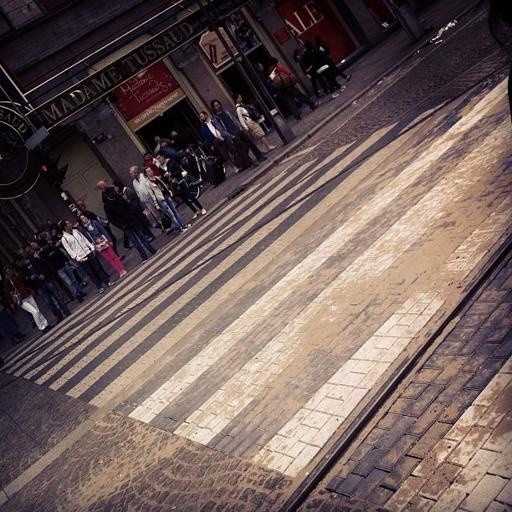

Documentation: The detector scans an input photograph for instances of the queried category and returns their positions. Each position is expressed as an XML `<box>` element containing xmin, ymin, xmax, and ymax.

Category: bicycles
<box><xmin>177</xmin><ymin>142</ymin><xmax>226</xmax><ymax>201</ymax></box>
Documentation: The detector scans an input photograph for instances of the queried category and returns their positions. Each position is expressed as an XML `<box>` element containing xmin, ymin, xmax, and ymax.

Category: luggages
<box><xmin>206</xmin><ymin>157</ymin><xmax>226</xmax><ymax>186</ymax></box>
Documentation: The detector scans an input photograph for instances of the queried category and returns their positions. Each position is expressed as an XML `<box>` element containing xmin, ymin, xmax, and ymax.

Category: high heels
<box><xmin>10</xmin><ymin>333</ymin><xmax>25</xmax><ymax>343</ymax></box>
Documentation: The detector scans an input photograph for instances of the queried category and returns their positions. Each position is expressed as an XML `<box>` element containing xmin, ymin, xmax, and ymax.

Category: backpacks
<box><xmin>236</xmin><ymin>104</ymin><xmax>260</xmax><ymax>121</ymax></box>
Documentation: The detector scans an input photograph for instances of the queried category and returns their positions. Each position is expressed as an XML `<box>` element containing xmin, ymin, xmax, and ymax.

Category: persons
<box><xmin>0</xmin><ymin>37</ymin><xmax>352</xmax><ymax>364</ymax></box>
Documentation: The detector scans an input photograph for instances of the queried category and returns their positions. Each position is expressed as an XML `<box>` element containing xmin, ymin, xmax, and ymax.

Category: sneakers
<box><xmin>124</xmin><ymin>236</ymin><xmax>160</xmax><ymax>264</ymax></box>
<box><xmin>297</xmin><ymin>102</ymin><xmax>319</xmax><ymax>121</ymax></box>
<box><xmin>317</xmin><ymin>74</ymin><xmax>352</xmax><ymax>100</ymax></box>
<box><xmin>57</xmin><ymin>281</ymin><xmax>88</xmax><ymax>323</ymax></box>
<box><xmin>32</xmin><ymin>321</ymin><xmax>54</xmax><ymax>333</ymax></box>
<box><xmin>252</xmin><ymin>146</ymin><xmax>277</xmax><ymax>167</ymax></box>
<box><xmin>179</xmin><ymin>208</ymin><xmax>207</xmax><ymax>232</ymax></box>
<box><xmin>98</xmin><ymin>269</ymin><xmax>127</xmax><ymax>294</ymax></box>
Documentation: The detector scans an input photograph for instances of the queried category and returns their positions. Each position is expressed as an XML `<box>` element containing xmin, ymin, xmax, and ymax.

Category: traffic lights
<box><xmin>31</xmin><ymin>142</ymin><xmax>69</xmax><ymax>180</ymax></box>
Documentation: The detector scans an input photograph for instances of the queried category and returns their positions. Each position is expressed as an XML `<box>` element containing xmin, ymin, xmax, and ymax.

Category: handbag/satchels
<box><xmin>96</xmin><ymin>240</ymin><xmax>109</xmax><ymax>252</ymax></box>
<box><xmin>142</xmin><ymin>208</ymin><xmax>159</xmax><ymax>228</ymax></box>
<box><xmin>271</xmin><ymin>72</ymin><xmax>298</xmax><ymax>89</ymax></box>
<box><xmin>160</xmin><ymin>215</ymin><xmax>171</xmax><ymax>229</ymax></box>
<box><xmin>233</xmin><ymin>152</ymin><xmax>251</xmax><ymax>167</ymax></box>
<box><xmin>10</xmin><ymin>291</ymin><xmax>22</xmax><ymax>305</ymax></box>
